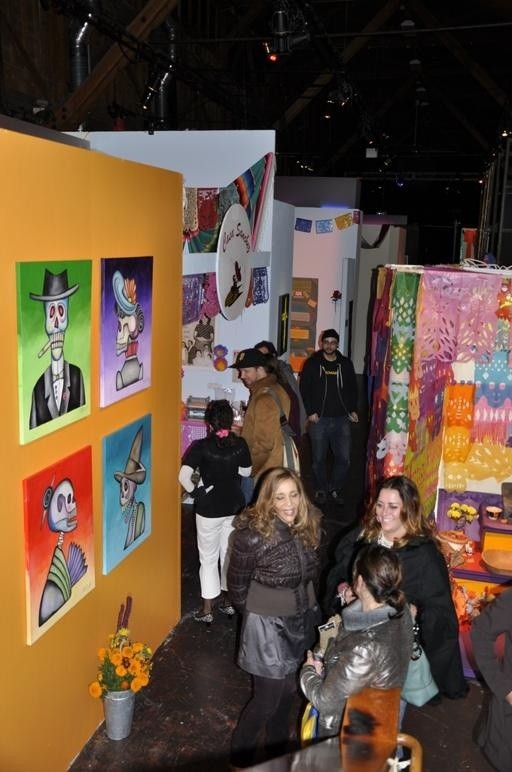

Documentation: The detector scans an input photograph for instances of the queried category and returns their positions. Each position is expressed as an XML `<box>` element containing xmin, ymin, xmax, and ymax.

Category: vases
<box><xmin>101</xmin><ymin>691</ymin><xmax>136</xmax><ymax>741</ymax></box>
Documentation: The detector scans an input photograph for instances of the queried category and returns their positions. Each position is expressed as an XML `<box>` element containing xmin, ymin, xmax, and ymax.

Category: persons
<box><xmin>226</xmin><ymin>466</ymin><xmax>453</xmax><ymax>767</ymax></box>
<box><xmin>470</xmin><ymin>588</ymin><xmax>512</xmax><ymax>772</ymax></box>
<box><xmin>177</xmin><ymin>329</ymin><xmax>359</xmax><ymax>626</ymax></box>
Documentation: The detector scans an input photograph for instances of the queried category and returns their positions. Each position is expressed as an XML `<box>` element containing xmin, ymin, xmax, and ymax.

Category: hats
<box><xmin>225</xmin><ymin>348</ymin><xmax>268</xmax><ymax>369</ymax></box>
<box><xmin>112</xmin><ymin>270</ymin><xmax>139</xmax><ymax>315</ymax></box>
<box><xmin>321</xmin><ymin>329</ymin><xmax>339</xmax><ymax>341</ymax></box>
<box><xmin>29</xmin><ymin>267</ymin><xmax>80</xmax><ymax>304</ymax></box>
<box><xmin>113</xmin><ymin>424</ymin><xmax>148</xmax><ymax>485</ymax></box>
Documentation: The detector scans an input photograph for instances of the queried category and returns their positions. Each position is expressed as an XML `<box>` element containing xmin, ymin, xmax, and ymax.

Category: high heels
<box><xmin>192</xmin><ymin>610</ymin><xmax>214</xmax><ymax>626</ymax></box>
<box><xmin>218</xmin><ymin>604</ymin><xmax>234</xmax><ymax>619</ymax></box>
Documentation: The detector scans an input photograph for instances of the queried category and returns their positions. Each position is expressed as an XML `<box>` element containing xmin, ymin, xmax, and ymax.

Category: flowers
<box><xmin>447</xmin><ymin>502</ymin><xmax>477</xmax><ymax>532</ymax></box>
<box><xmin>88</xmin><ymin>594</ymin><xmax>155</xmax><ymax>699</ymax></box>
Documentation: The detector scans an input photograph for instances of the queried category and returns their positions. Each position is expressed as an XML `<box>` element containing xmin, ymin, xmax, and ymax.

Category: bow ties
<box><xmin>53</xmin><ymin>369</ymin><xmax>65</xmax><ymax>384</ymax></box>
